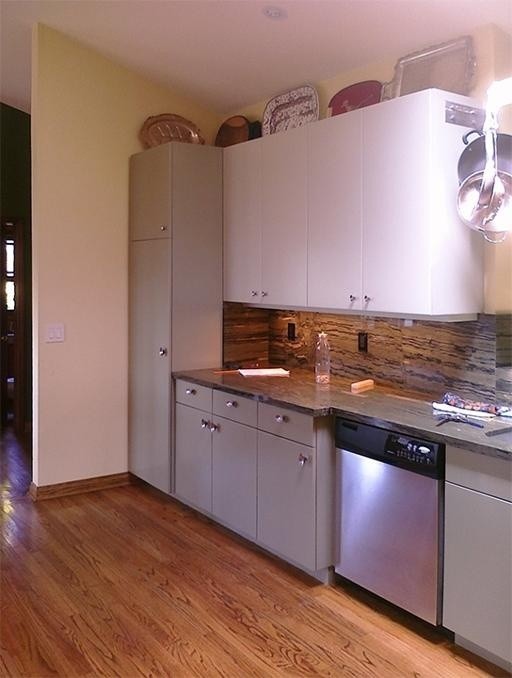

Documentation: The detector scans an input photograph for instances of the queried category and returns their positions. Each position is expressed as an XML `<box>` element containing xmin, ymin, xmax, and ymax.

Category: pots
<box><xmin>456</xmin><ymin>128</ymin><xmax>512</xmax><ymax>244</ymax></box>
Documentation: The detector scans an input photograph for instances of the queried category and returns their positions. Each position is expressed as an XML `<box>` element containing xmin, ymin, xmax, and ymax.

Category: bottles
<box><xmin>315</xmin><ymin>333</ymin><xmax>330</xmax><ymax>384</ymax></box>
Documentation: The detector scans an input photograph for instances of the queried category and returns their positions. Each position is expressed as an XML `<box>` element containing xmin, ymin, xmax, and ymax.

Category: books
<box><xmin>236</xmin><ymin>367</ymin><xmax>291</xmax><ymax>377</ymax></box>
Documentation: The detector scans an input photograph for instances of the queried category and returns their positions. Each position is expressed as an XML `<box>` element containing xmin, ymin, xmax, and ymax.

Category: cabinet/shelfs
<box><xmin>258</xmin><ymin>401</ymin><xmax>333</xmax><ymax>588</ymax></box>
<box><xmin>222</xmin><ymin>124</ymin><xmax>307</xmax><ymax>311</ymax></box>
<box><xmin>308</xmin><ymin>88</ymin><xmax>486</xmax><ymax>323</ymax></box>
<box><xmin>127</xmin><ymin>141</ymin><xmax>223</xmax><ymax>496</ymax></box>
<box><xmin>440</xmin><ymin>443</ymin><xmax>511</xmax><ymax>672</ymax></box>
<box><xmin>175</xmin><ymin>378</ymin><xmax>258</xmax><ymax>546</ymax></box>
